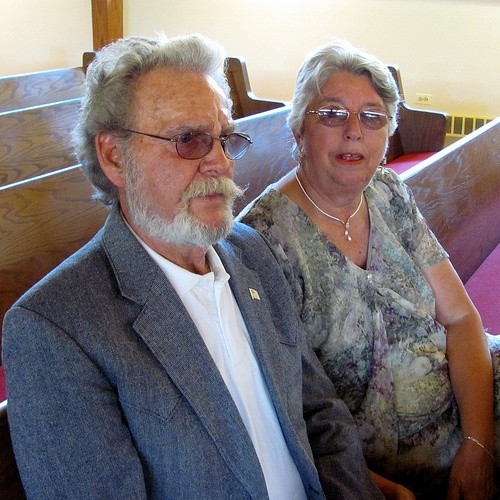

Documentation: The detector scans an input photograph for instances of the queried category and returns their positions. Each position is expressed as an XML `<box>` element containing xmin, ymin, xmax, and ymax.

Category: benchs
<box><xmin>0</xmin><ymin>51</ymin><xmax>500</xmax><ymax>500</ymax></box>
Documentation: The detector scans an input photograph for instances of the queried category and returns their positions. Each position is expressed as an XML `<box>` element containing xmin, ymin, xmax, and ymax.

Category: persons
<box><xmin>236</xmin><ymin>44</ymin><xmax>500</xmax><ymax>499</ymax></box>
<box><xmin>1</xmin><ymin>36</ymin><xmax>383</xmax><ymax>498</ymax></box>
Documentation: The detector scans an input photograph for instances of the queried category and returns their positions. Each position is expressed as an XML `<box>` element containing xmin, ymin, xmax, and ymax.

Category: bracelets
<box><xmin>463</xmin><ymin>436</ymin><xmax>496</xmax><ymax>464</ymax></box>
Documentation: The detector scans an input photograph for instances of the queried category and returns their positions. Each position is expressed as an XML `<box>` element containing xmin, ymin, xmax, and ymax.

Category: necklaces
<box><xmin>295</xmin><ymin>167</ymin><xmax>363</xmax><ymax>242</ymax></box>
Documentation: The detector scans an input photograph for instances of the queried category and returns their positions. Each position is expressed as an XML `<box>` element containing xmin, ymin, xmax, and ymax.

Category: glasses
<box><xmin>304</xmin><ymin>105</ymin><xmax>392</xmax><ymax>130</ymax></box>
<box><xmin>115</xmin><ymin>126</ymin><xmax>251</xmax><ymax>159</ymax></box>
